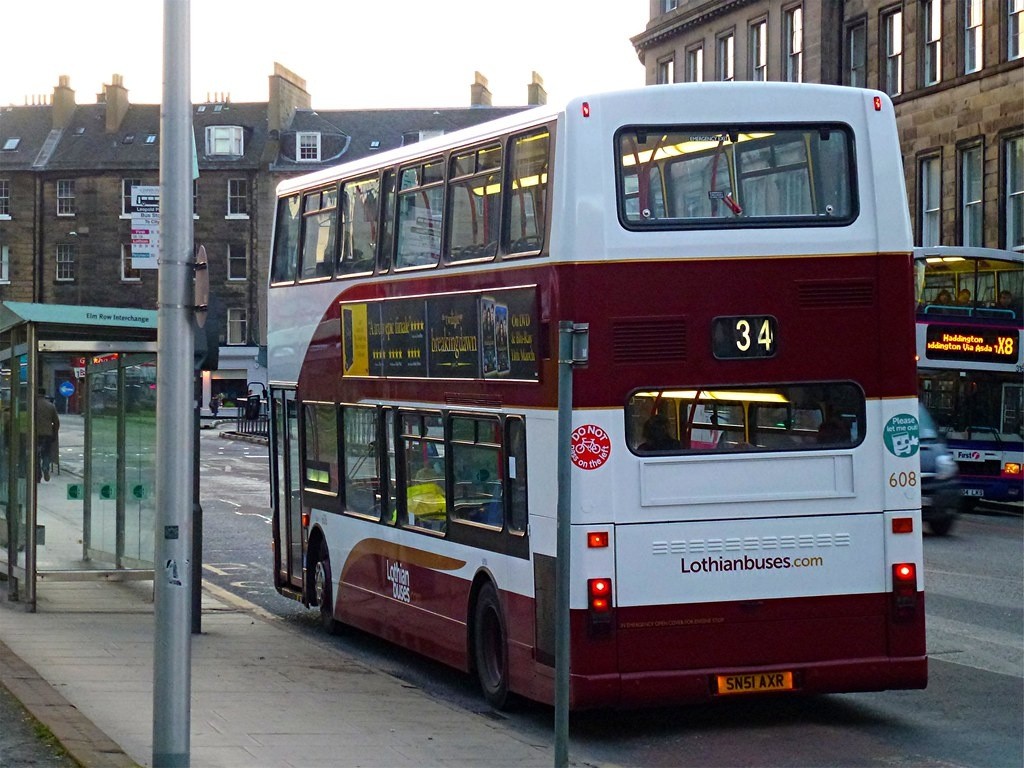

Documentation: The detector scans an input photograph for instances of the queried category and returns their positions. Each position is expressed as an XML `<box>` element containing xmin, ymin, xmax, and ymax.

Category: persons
<box><xmin>954</xmin><ymin>288</ymin><xmax>972</xmax><ymax>307</ymax></box>
<box><xmin>934</xmin><ymin>290</ymin><xmax>952</xmax><ymax>306</ymax></box>
<box><xmin>211</xmin><ymin>394</ymin><xmax>220</xmax><ymax>416</ymax></box>
<box><xmin>425</xmin><ymin>427</ymin><xmax>438</xmax><ymax>456</ymax></box>
<box><xmin>995</xmin><ymin>290</ymin><xmax>1014</xmax><ymax>310</ymax></box>
<box><xmin>392</xmin><ymin>467</ymin><xmax>446</xmax><ymax>523</ymax></box>
<box><xmin>28</xmin><ymin>388</ymin><xmax>60</xmax><ymax>483</ymax></box>
<box><xmin>638</xmin><ymin>414</ymin><xmax>681</xmax><ymax>451</ymax></box>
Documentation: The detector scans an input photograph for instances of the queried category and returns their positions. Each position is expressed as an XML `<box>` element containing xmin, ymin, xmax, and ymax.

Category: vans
<box><xmin>729</xmin><ymin>403</ymin><xmax>959</xmax><ymax>535</ymax></box>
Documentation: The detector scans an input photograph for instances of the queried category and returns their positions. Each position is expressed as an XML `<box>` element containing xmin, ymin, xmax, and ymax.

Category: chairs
<box><xmin>347</xmin><ymin>479</ymin><xmax>519</xmax><ymax>533</ymax></box>
<box><xmin>290</xmin><ymin>232</ymin><xmax>542</xmax><ymax>278</ymax></box>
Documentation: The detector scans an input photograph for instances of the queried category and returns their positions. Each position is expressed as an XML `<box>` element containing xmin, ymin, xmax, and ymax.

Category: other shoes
<box><xmin>44</xmin><ymin>468</ymin><xmax>50</xmax><ymax>481</ymax></box>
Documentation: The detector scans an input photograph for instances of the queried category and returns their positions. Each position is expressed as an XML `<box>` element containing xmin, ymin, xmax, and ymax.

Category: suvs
<box><xmin>0</xmin><ymin>366</ymin><xmax>157</xmax><ymax>413</ymax></box>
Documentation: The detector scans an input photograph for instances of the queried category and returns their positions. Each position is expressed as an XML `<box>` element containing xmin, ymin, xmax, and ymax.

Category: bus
<box><xmin>245</xmin><ymin>81</ymin><xmax>929</xmax><ymax>711</ymax></box>
<box><xmin>688</xmin><ymin>246</ymin><xmax>1024</xmax><ymax>510</ymax></box>
